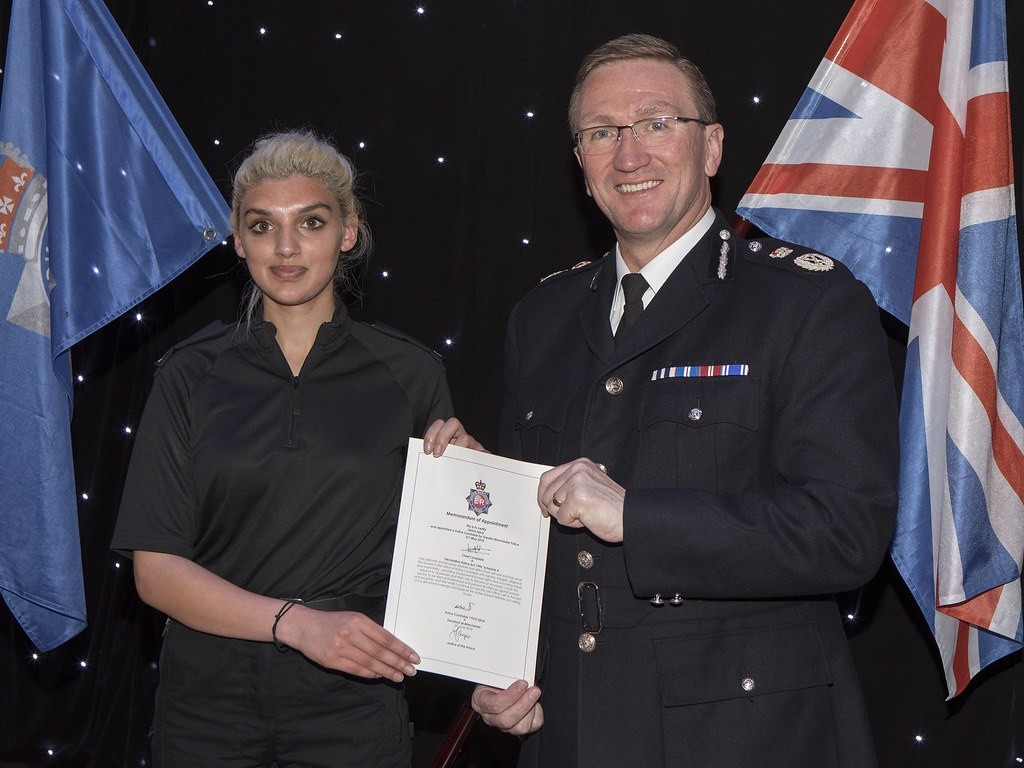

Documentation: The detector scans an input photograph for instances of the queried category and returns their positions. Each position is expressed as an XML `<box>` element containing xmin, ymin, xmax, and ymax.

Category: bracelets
<box><xmin>272</xmin><ymin>598</ymin><xmax>304</xmax><ymax>652</ymax></box>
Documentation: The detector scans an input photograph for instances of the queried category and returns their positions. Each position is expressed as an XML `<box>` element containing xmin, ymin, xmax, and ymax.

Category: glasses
<box><xmin>573</xmin><ymin>115</ymin><xmax>709</xmax><ymax>155</ymax></box>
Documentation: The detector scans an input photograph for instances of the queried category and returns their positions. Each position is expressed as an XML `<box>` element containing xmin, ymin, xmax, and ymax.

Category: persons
<box><xmin>111</xmin><ymin>131</ymin><xmax>490</xmax><ymax>768</ymax></box>
<box><xmin>471</xmin><ymin>34</ymin><xmax>900</xmax><ymax>768</ymax></box>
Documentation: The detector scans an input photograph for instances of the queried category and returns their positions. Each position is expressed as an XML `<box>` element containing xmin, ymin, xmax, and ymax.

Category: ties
<box><xmin>614</xmin><ymin>273</ymin><xmax>650</xmax><ymax>352</ymax></box>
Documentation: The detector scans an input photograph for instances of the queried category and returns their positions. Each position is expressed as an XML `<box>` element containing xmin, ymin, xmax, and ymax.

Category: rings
<box><xmin>552</xmin><ymin>493</ymin><xmax>562</xmax><ymax>507</ymax></box>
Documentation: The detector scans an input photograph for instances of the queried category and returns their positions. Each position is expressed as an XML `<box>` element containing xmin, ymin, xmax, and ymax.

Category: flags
<box><xmin>0</xmin><ymin>0</ymin><xmax>234</xmax><ymax>654</ymax></box>
<box><xmin>734</xmin><ymin>1</ymin><xmax>1024</xmax><ymax>702</ymax></box>
